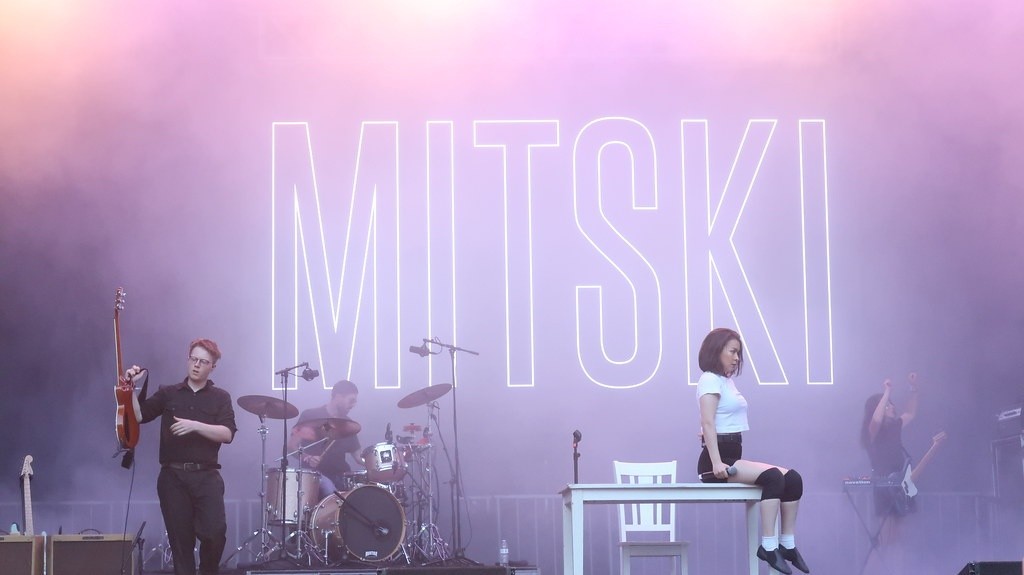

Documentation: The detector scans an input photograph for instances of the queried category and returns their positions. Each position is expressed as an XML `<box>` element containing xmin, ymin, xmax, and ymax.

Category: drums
<box><xmin>266</xmin><ymin>468</ymin><xmax>322</xmax><ymax>526</ymax></box>
<box><xmin>343</xmin><ymin>470</ymin><xmax>406</xmax><ymax>504</ymax></box>
<box><xmin>362</xmin><ymin>442</ymin><xmax>408</xmax><ymax>482</ymax></box>
<box><xmin>309</xmin><ymin>485</ymin><xmax>407</xmax><ymax>564</ymax></box>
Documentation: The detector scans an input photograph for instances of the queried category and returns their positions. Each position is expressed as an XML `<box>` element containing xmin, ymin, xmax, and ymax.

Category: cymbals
<box><xmin>292</xmin><ymin>418</ymin><xmax>362</xmax><ymax>440</ymax></box>
<box><xmin>398</xmin><ymin>383</ymin><xmax>452</xmax><ymax>409</ymax></box>
<box><xmin>237</xmin><ymin>395</ymin><xmax>299</xmax><ymax>419</ymax></box>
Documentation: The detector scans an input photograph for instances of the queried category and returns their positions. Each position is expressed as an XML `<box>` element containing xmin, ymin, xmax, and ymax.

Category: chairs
<box><xmin>612</xmin><ymin>460</ymin><xmax>690</xmax><ymax>575</ymax></box>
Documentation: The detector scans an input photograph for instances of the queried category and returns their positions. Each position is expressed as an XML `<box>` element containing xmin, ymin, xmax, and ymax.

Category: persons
<box><xmin>125</xmin><ymin>339</ymin><xmax>237</xmax><ymax>575</ymax></box>
<box><xmin>288</xmin><ymin>381</ymin><xmax>364</xmax><ymax>503</ymax></box>
<box><xmin>698</xmin><ymin>327</ymin><xmax>810</xmax><ymax>575</ymax></box>
<box><xmin>860</xmin><ymin>371</ymin><xmax>919</xmax><ymax>548</ymax></box>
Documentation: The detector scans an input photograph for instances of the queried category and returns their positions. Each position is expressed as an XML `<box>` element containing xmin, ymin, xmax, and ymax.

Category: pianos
<box><xmin>840</xmin><ymin>475</ymin><xmax>896</xmax><ymax>575</ymax></box>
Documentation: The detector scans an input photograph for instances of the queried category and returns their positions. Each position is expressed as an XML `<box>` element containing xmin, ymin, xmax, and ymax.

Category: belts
<box><xmin>718</xmin><ymin>434</ymin><xmax>742</xmax><ymax>443</ymax></box>
<box><xmin>162</xmin><ymin>462</ymin><xmax>222</xmax><ymax>471</ymax></box>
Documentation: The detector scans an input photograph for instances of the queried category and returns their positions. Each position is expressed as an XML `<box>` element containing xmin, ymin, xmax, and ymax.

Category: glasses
<box><xmin>188</xmin><ymin>356</ymin><xmax>214</xmax><ymax>364</ymax></box>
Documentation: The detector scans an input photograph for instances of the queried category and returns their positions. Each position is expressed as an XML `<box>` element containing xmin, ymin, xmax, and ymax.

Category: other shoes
<box><xmin>757</xmin><ymin>544</ymin><xmax>792</xmax><ymax>574</ymax></box>
<box><xmin>779</xmin><ymin>544</ymin><xmax>809</xmax><ymax>573</ymax></box>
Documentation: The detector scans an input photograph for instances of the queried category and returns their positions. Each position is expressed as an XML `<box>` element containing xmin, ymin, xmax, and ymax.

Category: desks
<box><xmin>556</xmin><ymin>483</ymin><xmax>762</xmax><ymax>575</ymax></box>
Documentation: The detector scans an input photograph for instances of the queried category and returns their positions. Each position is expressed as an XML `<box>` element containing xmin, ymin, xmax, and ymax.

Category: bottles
<box><xmin>498</xmin><ymin>539</ymin><xmax>509</xmax><ymax>567</ymax></box>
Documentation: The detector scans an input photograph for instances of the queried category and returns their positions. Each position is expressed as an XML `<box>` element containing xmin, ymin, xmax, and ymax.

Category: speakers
<box><xmin>991</xmin><ymin>435</ymin><xmax>1024</xmax><ymax>560</ymax></box>
<box><xmin>46</xmin><ymin>534</ymin><xmax>137</xmax><ymax>575</ymax></box>
<box><xmin>957</xmin><ymin>561</ymin><xmax>1024</xmax><ymax>575</ymax></box>
<box><xmin>0</xmin><ymin>534</ymin><xmax>44</xmax><ymax>575</ymax></box>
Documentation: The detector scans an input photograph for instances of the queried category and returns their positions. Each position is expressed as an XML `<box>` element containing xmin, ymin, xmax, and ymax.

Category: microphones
<box><xmin>409</xmin><ymin>345</ymin><xmax>437</xmax><ymax>356</ymax></box>
<box><xmin>301</xmin><ymin>370</ymin><xmax>319</xmax><ymax>377</ymax></box>
<box><xmin>378</xmin><ymin>527</ymin><xmax>390</xmax><ymax>536</ymax></box>
<box><xmin>385</xmin><ymin>424</ymin><xmax>391</xmax><ymax>440</ymax></box>
<box><xmin>428</xmin><ymin>402</ymin><xmax>435</xmax><ymax>419</ymax></box>
<box><xmin>699</xmin><ymin>466</ymin><xmax>738</xmax><ymax>480</ymax></box>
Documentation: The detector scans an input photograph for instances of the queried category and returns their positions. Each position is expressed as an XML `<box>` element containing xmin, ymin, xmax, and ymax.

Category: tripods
<box><xmin>220</xmin><ymin>362</ymin><xmax>336</xmax><ymax>570</ymax></box>
<box><xmin>391</xmin><ymin>339</ymin><xmax>486</xmax><ymax>568</ymax></box>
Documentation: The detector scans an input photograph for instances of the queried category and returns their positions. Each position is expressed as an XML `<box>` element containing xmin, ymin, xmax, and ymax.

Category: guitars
<box><xmin>9</xmin><ymin>454</ymin><xmax>47</xmax><ymax>575</ymax></box>
<box><xmin>113</xmin><ymin>287</ymin><xmax>140</xmax><ymax>450</ymax></box>
<box><xmin>888</xmin><ymin>430</ymin><xmax>947</xmax><ymax>514</ymax></box>
